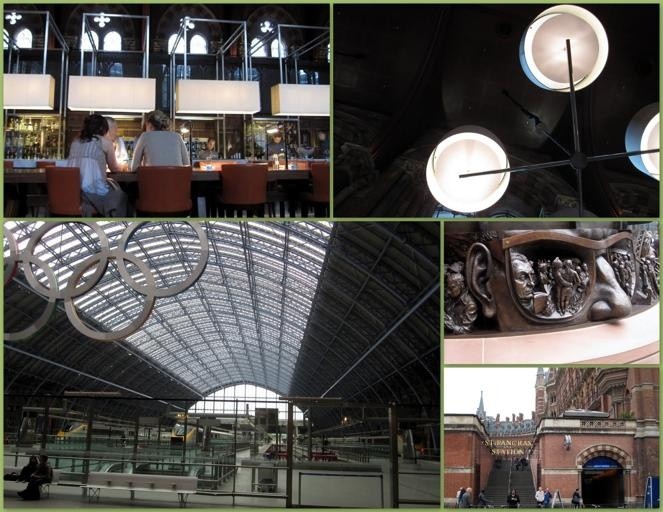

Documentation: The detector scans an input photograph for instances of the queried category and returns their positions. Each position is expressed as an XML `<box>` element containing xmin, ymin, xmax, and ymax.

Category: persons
<box><xmin>450</xmin><ymin>229</ymin><xmax>632</xmax><ymax>332</ymax></box>
<box><xmin>268</xmin><ymin>132</ymin><xmax>291</xmax><ymax>159</ymax></box>
<box><xmin>197</xmin><ymin>137</ymin><xmax>219</xmax><ymax>159</ymax></box>
<box><xmin>510</xmin><ymin>252</ymin><xmax>535</xmax><ymax>312</ymax></box>
<box><xmin>16</xmin><ymin>456</ymin><xmax>38</xmax><ymax>483</ymax></box>
<box><xmin>65</xmin><ymin>115</ymin><xmax>128</xmax><ymax>217</ymax></box>
<box><xmin>102</xmin><ymin>117</ymin><xmax>128</xmax><ymax>172</ymax></box>
<box><xmin>444</xmin><ymin>272</ymin><xmax>478</xmax><ymax>335</ymax></box>
<box><xmin>456</xmin><ymin>457</ymin><xmax>582</xmax><ymax>508</ymax></box>
<box><xmin>131</xmin><ymin>110</ymin><xmax>190</xmax><ymax>172</ymax></box>
<box><xmin>17</xmin><ymin>455</ymin><xmax>51</xmax><ymax>500</ymax></box>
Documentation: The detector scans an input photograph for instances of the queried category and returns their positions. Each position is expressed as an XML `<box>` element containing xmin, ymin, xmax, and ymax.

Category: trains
<box><xmin>56</xmin><ymin>420</ymin><xmax>171</xmax><ymax>447</ymax></box>
<box><xmin>172</xmin><ymin>419</ymin><xmax>254</xmax><ymax>452</ymax></box>
<box><xmin>301</xmin><ymin>432</ymin><xmax>403</xmax><ymax>458</ymax></box>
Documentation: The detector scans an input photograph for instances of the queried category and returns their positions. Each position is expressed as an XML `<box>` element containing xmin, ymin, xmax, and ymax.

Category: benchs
<box><xmin>81</xmin><ymin>472</ymin><xmax>198</xmax><ymax>508</ymax></box>
<box><xmin>3</xmin><ymin>466</ymin><xmax>59</xmax><ymax>498</ymax></box>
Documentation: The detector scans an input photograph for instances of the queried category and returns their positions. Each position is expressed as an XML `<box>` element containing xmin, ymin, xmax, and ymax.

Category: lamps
<box><xmin>425</xmin><ymin>5</ymin><xmax>661</xmax><ymax>215</ymax></box>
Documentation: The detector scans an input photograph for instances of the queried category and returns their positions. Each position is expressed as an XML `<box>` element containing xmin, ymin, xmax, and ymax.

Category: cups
<box><xmin>290</xmin><ymin>157</ymin><xmax>297</xmax><ymax>169</ymax></box>
<box><xmin>121</xmin><ymin>159</ymin><xmax>129</xmax><ymax>172</ymax></box>
<box><xmin>205</xmin><ymin>157</ymin><xmax>212</xmax><ymax>170</ymax></box>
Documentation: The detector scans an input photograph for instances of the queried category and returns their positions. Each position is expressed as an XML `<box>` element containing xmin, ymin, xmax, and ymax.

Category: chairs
<box><xmin>221</xmin><ymin>164</ymin><xmax>267</xmax><ymax>204</ymax></box>
<box><xmin>136</xmin><ymin>166</ymin><xmax>193</xmax><ymax>212</ymax></box>
<box><xmin>46</xmin><ymin>167</ymin><xmax>87</xmax><ymax>214</ymax></box>
<box><xmin>305</xmin><ymin>163</ymin><xmax>332</xmax><ymax>204</ymax></box>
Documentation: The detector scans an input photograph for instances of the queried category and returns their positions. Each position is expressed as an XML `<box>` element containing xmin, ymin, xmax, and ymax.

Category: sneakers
<box><xmin>15</xmin><ymin>479</ymin><xmax>26</xmax><ymax>482</ymax></box>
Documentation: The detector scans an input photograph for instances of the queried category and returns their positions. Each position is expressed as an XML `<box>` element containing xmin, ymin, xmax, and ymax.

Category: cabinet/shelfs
<box><xmin>5</xmin><ymin>114</ymin><xmax>65</xmax><ymax>159</ymax></box>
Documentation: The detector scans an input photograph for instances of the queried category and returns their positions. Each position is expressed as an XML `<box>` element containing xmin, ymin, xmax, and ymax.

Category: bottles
<box><xmin>272</xmin><ymin>153</ymin><xmax>279</xmax><ymax>170</ymax></box>
<box><xmin>5</xmin><ymin>117</ymin><xmax>62</xmax><ymax>161</ymax></box>
